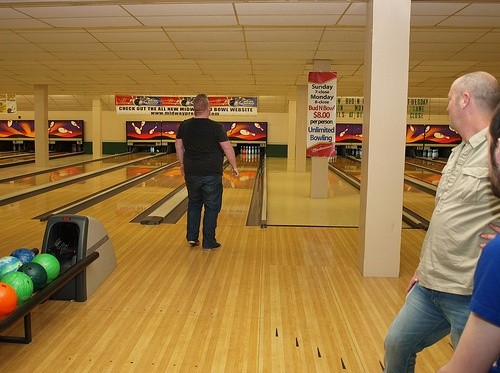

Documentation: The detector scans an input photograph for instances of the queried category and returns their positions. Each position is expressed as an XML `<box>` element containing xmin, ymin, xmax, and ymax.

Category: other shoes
<box><xmin>188</xmin><ymin>239</ymin><xmax>200</xmax><ymax>246</ymax></box>
<box><xmin>203</xmin><ymin>243</ymin><xmax>221</xmax><ymax>250</ymax></box>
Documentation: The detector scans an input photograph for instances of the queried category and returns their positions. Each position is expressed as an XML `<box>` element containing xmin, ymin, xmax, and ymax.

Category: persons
<box><xmin>175</xmin><ymin>93</ymin><xmax>239</xmax><ymax>250</ymax></box>
<box><xmin>383</xmin><ymin>71</ymin><xmax>500</xmax><ymax>373</ymax></box>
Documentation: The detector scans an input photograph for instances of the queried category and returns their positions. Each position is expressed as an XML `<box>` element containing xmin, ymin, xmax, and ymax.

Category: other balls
<box><xmin>0</xmin><ymin>247</ymin><xmax>61</xmax><ymax>318</ymax></box>
